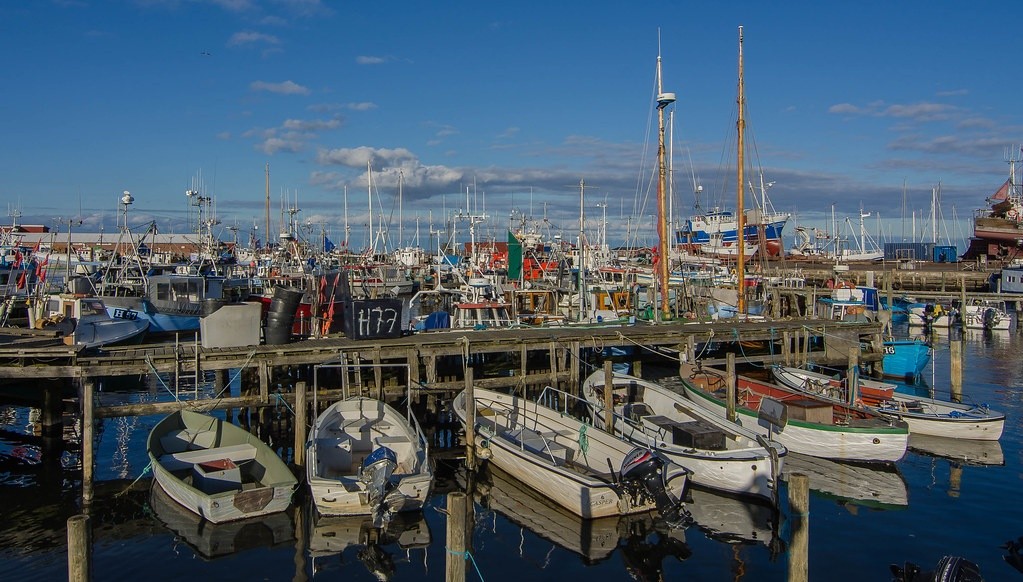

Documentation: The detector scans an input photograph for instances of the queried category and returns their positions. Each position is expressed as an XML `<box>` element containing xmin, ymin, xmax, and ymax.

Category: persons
<box><xmin>0</xmin><ymin>299</ymin><xmax>10</xmax><ymax>329</ymax></box>
<box><xmin>408</xmin><ymin>320</ymin><xmax>413</xmax><ymax>335</ymax></box>
<box><xmin>620</xmin><ymin>296</ymin><xmax>626</xmax><ymax>307</ymax></box>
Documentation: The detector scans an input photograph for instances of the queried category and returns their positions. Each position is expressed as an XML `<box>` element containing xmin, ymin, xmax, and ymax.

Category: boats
<box><xmin>863</xmin><ymin>339</ymin><xmax>934</xmax><ymax>384</ymax></box>
<box><xmin>453</xmin><ymin>456</ymin><xmax>694</xmax><ymax>582</ymax></box>
<box><xmin>778</xmin><ymin>448</ymin><xmax>910</xmax><ymax>510</ymax></box>
<box><xmin>764</xmin><ymin>359</ymin><xmax>1007</xmax><ymax>442</ymax></box>
<box><xmin>452</xmin><ymin>384</ymin><xmax>690</xmax><ymax>521</ymax></box>
<box><xmin>145</xmin><ymin>408</ymin><xmax>299</xmax><ymax>524</ymax></box>
<box><xmin>677</xmin><ymin>481</ymin><xmax>785</xmax><ymax>564</ymax></box>
<box><xmin>307</xmin><ymin>496</ymin><xmax>430</xmax><ymax>582</ymax></box>
<box><xmin>677</xmin><ymin>356</ymin><xmax>911</xmax><ymax>465</ymax></box>
<box><xmin>583</xmin><ymin>367</ymin><xmax>789</xmax><ymax>510</ymax></box>
<box><xmin>144</xmin><ymin>475</ymin><xmax>295</xmax><ymax>562</ymax></box>
<box><xmin>305</xmin><ymin>362</ymin><xmax>435</xmax><ymax>527</ymax></box>
<box><xmin>904</xmin><ymin>432</ymin><xmax>1006</xmax><ymax>467</ymax></box>
<box><xmin>0</xmin><ymin>19</ymin><xmax>1012</xmax><ymax>335</ymax></box>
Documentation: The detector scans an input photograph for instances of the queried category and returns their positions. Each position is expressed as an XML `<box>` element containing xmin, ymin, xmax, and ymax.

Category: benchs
<box><xmin>326</xmin><ymin>418</ymin><xmax>397</xmax><ymax>452</ymax></box>
<box><xmin>159</xmin><ymin>442</ymin><xmax>257</xmax><ymax>471</ymax></box>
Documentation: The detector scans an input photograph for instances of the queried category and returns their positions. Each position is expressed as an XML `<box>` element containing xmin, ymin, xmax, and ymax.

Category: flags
<box><xmin>327</xmin><ymin>277</ymin><xmax>338</xmax><ymax>320</ymax></box>
<box><xmin>36</xmin><ymin>263</ymin><xmax>41</xmax><ymax>277</ymax></box>
<box><xmin>40</xmin><ymin>268</ymin><xmax>46</xmax><ymax>283</ymax></box>
<box><xmin>41</xmin><ymin>254</ymin><xmax>48</xmax><ymax>266</ymax></box>
<box><xmin>17</xmin><ymin>271</ymin><xmax>26</xmax><ymax>288</ymax></box>
<box><xmin>14</xmin><ymin>237</ymin><xmax>22</xmax><ymax>247</ymax></box>
<box><xmin>138</xmin><ymin>241</ymin><xmax>150</xmax><ymax>255</ymax></box>
<box><xmin>12</xmin><ymin>250</ymin><xmax>23</xmax><ymax>267</ymax></box>
<box><xmin>34</xmin><ymin>239</ymin><xmax>40</xmax><ymax>252</ymax></box>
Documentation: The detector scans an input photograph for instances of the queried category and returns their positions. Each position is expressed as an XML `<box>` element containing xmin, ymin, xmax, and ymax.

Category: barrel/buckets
<box><xmin>262</xmin><ymin>284</ymin><xmax>307</xmax><ymax>345</ymax></box>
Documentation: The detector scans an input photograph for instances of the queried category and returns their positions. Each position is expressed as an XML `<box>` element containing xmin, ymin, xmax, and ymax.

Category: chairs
<box><xmin>629</xmin><ymin>405</ymin><xmax>651</xmax><ymax>425</ymax></box>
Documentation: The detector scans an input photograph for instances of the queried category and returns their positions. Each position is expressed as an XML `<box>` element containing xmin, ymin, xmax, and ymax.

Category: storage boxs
<box><xmin>932</xmin><ymin>245</ymin><xmax>958</xmax><ymax>263</ymax></box>
<box><xmin>828</xmin><ymin>378</ymin><xmax>898</xmax><ymax>406</ymax></box>
<box><xmin>884</xmin><ymin>242</ymin><xmax>937</xmax><ymax>261</ymax></box>
<box><xmin>464</xmin><ymin>242</ymin><xmax>544</xmax><ymax>255</ymax></box>
